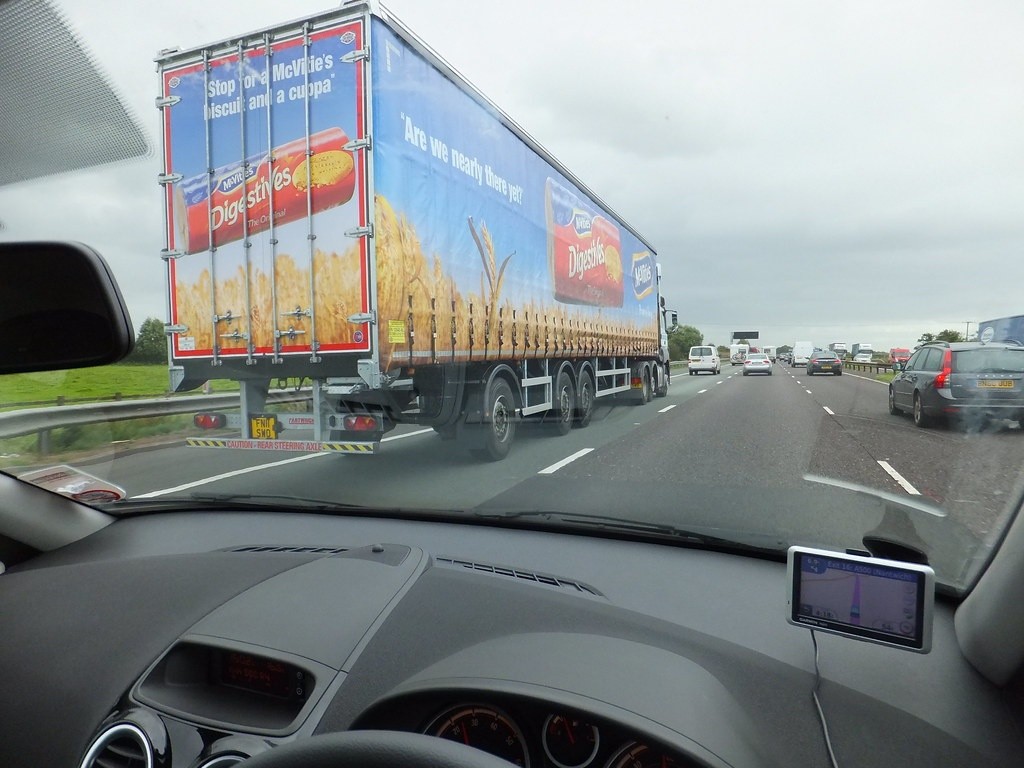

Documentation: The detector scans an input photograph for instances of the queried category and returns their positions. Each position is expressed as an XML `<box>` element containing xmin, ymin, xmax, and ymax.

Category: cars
<box><xmin>743</xmin><ymin>353</ymin><xmax>773</xmax><ymax>376</ymax></box>
<box><xmin>779</xmin><ymin>353</ymin><xmax>786</xmax><ymax>361</ymax></box>
<box><xmin>784</xmin><ymin>353</ymin><xmax>788</xmax><ymax>362</ymax></box>
<box><xmin>688</xmin><ymin>346</ymin><xmax>721</xmax><ymax>375</ymax></box>
<box><xmin>807</xmin><ymin>352</ymin><xmax>842</xmax><ymax>376</ymax></box>
<box><xmin>788</xmin><ymin>352</ymin><xmax>793</xmax><ymax>364</ymax></box>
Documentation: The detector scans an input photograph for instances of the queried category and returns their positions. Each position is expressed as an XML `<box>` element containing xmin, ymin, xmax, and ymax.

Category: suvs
<box><xmin>888</xmin><ymin>340</ymin><xmax>1024</xmax><ymax>430</ymax></box>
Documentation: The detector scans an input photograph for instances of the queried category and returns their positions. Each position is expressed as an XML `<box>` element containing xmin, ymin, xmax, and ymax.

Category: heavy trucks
<box><xmin>153</xmin><ymin>0</ymin><xmax>679</xmax><ymax>464</ymax></box>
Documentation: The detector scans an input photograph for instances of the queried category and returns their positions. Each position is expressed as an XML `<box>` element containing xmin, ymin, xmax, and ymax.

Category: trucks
<box><xmin>792</xmin><ymin>341</ymin><xmax>813</xmax><ymax>368</ymax></box>
<box><xmin>729</xmin><ymin>344</ymin><xmax>749</xmax><ymax>366</ymax></box>
<box><xmin>889</xmin><ymin>347</ymin><xmax>910</xmax><ymax>363</ymax></box>
<box><xmin>763</xmin><ymin>346</ymin><xmax>776</xmax><ymax>363</ymax></box>
<box><xmin>829</xmin><ymin>343</ymin><xmax>847</xmax><ymax>360</ymax></box>
<box><xmin>852</xmin><ymin>343</ymin><xmax>872</xmax><ymax>362</ymax></box>
